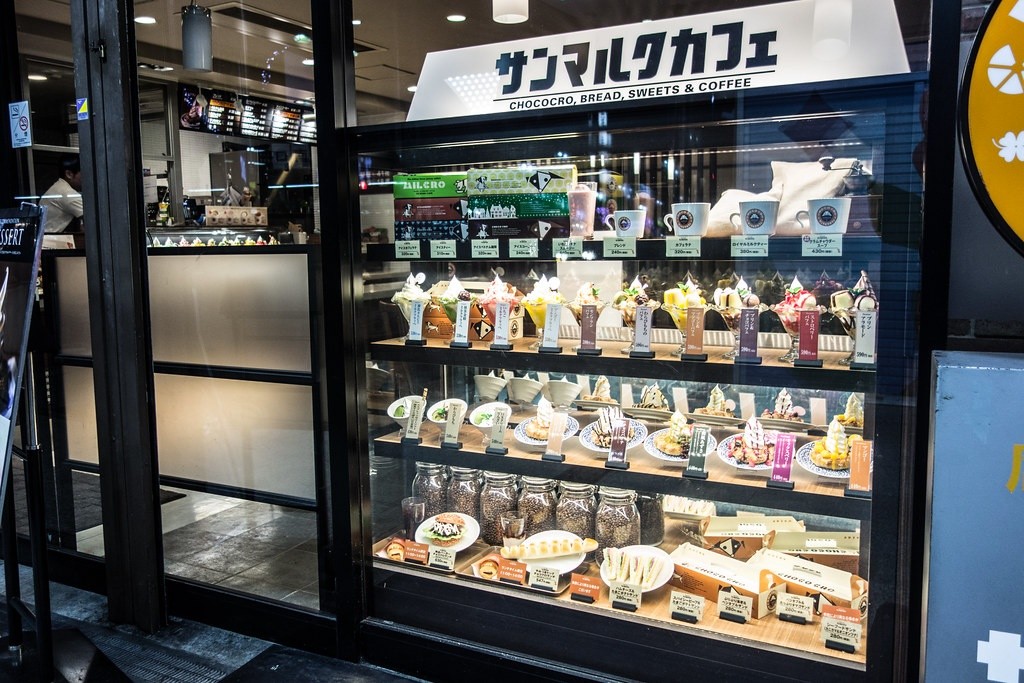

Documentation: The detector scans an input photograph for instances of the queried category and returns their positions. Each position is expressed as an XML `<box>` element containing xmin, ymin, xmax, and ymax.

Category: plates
<box><xmin>716</xmin><ymin>431</ymin><xmax>795</xmax><ymax>470</ymax></box>
<box><xmin>579</xmin><ymin>419</ymin><xmax>649</xmax><ymax>453</ymax></box>
<box><xmin>643</xmin><ymin>426</ymin><xmax>718</xmax><ymax>461</ymax></box>
<box><xmin>415</xmin><ymin>512</ymin><xmax>480</xmax><ymax>553</ymax></box>
<box><xmin>573</xmin><ymin>399</ymin><xmax>864</xmax><ymax>436</ymax></box>
<box><xmin>796</xmin><ymin>440</ymin><xmax>874</xmax><ymax>478</ymax></box>
<box><xmin>599</xmin><ymin>545</ymin><xmax>674</xmax><ymax>592</ymax></box>
<box><xmin>513</xmin><ymin>412</ymin><xmax>580</xmax><ymax>445</ymax></box>
<box><xmin>518</xmin><ymin>530</ymin><xmax>586</xmax><ymax>575</ymax></box>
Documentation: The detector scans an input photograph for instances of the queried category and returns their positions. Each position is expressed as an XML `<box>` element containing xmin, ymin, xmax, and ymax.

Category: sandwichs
<box><xmin>602</xmin><ymin>546</ymin><xmax>664</xmax><ymax>589</ymax></box>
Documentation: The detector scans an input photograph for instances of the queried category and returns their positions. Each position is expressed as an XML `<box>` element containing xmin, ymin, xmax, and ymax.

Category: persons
<box><xmin>38</xmin><ymin>153</ymin><xmax>84</xmax><ymax>234</ymax></box>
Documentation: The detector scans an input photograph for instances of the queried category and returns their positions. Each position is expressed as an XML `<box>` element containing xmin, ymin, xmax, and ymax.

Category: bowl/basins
<box><xmin>473</xmin><ymin>375</ymin><xmax>582</xmax><ymax>407</ymax></box>
<box><xmin>426</xmin><ymin>398</ymin><xmax>467</xmax><ymax>435</ymax></box>
<box><xmin>386</xmin><ymin>395</ymin><xmax>427</xmax><ymax>429</ymax></box>
<box><xmin>469</xmin><ymin>401</ymin><xmax>512</xmax><ymax>439</ymax></box>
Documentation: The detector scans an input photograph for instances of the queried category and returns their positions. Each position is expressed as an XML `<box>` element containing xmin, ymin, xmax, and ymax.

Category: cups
<box><xmin>402</xmin><ymin>497</ymin><xmax>427</xmax><ymax>542</ymax></box>
<box><xmin>729</xmin><ymin>200</ymin><xmax>780</xmax><ymax>235</ymax></box>
<box><xmin>664</xmin><ymin>202</ymin><xmax>711</xmax><ymax>236</ymax></box>
<box><xmin>567</xmin><ymin>181</ymin><xmax>598</xmax><ymax>240</ymax></box>
<box><xmin>501</xmin><ymin>511</ymin><xmax>525</xmax><ymax>548</ymax></box>
<box><xmin>796</xmin><ymin>199</ymin><xmax>852</xmax><ymax>235</ymax></box>
<box><xmin>605</xmin><ymin>210</ymin><xmax>647</xmax><ymax>238</ymax></box>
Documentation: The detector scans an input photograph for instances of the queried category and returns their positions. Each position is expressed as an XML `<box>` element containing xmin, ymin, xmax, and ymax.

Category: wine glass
<box><xmin>520</xmin><ymin>299</ymin><xmax>880</xmax><ymax>366</ymax></box>
<box><xmin>393</xmin><ymin>300</ymin><xmax>519</xmax><ymax>348</ymax></box>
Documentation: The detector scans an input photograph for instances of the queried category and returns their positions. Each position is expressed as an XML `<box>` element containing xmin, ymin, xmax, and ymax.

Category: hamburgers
<box><xmin>422</xmin><ymin>513</ymin><xmax>465</xmax><ymax>547</ymax></box>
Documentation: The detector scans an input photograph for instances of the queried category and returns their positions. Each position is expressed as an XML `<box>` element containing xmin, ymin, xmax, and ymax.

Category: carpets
<box><xmin>219</xmin><ymin>644</ymin><xmax>423</xmax><ymax>683</ymax></box>
<box><xmin>11</xmin><ymin>457</ymin><xmax>186</xmax><ymax>536</ymax></box>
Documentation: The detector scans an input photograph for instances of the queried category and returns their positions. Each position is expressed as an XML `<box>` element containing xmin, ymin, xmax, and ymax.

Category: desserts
<box><xmin>390</xmin><ymin>270</ymin><xmax>881</xmax><ymax>472</ymax></box>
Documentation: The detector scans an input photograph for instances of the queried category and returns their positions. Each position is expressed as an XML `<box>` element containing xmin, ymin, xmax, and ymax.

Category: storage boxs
<box><xmin>661</xmin><ymin>502</ymin><xmax>870</xmax><ymax>620</ymax></box>
<box><xmin>422</xmin><ymin>280</ymin><xmax>525</xmax><ymax>340</ymax></box>
<box><xmin>393</xmin><ymin>164</ymin><xmax>577</xmax><ymax>239</ymax></box>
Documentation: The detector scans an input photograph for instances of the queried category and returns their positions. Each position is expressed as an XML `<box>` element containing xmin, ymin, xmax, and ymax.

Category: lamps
<box><xmin>235</xmin><ymin>94</ymin><xmax>244</xmax><ymax>113</ymax></box>
<box><xmin>174</xmin><ymin>0</ymin><xmax>212</xmax><ymax>72</ymax></box>
<box><xmin>196</xmin><ymin>87</ymin><xmax>207</xmax><ymax>106</ymax></box>
<box><xmin>493</xmin><ymin>1</ymin><xmax>529</xmax><ymax>24</ymax></box>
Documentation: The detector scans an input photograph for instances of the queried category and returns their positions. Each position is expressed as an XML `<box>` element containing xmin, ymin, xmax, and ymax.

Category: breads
<box><xmin>479</xmin><ymin>559</ymin><xmax>499</xmax><ymax>579</ymax></box>
<box><xmin>500</xmin><ymin>538</ymin><xmax>599</xmax><ymax>559</ymax></box>
<box><xmin>386</xmin><ymin>542</ymin><xmax>403</xmax><ymax>561</ymax></box>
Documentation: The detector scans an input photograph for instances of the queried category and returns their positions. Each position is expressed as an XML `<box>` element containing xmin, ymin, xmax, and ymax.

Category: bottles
<box><xmin>637</xmin><ymin>490</ymin><xmax>665</xmax><ymax>547</ymax></box>
<box><xmin>412</xmin><ymin>460</ymin><xmax>641</xmax><ymax>568</ymax></box>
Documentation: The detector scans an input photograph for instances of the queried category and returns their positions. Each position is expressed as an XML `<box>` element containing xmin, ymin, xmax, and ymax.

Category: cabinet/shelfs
<box><xmin>354</xmin><ymin>81</ymin><xmax>915</xmax><ymax>683</ymax></box>
